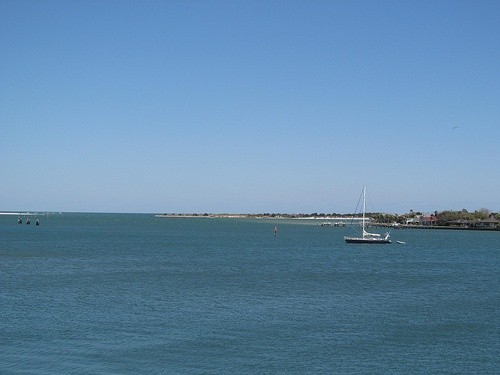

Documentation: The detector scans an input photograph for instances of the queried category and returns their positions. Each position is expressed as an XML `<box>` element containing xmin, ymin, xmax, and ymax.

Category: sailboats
<box><xmin>344</xmin><ymin>186</ymin><xmax>392</xmax><ymax>244</ymax></box>
